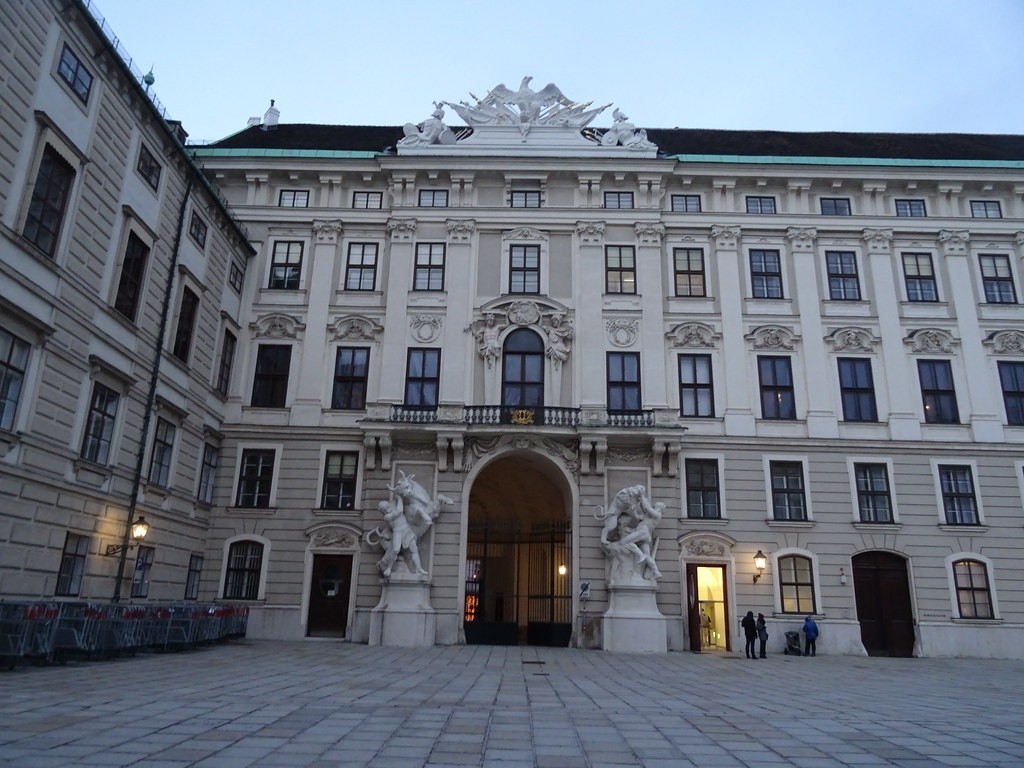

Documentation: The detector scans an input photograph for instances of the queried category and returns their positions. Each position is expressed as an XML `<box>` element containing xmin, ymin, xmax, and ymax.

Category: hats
<box><xmin>759</xmin><ymin>613</ymin><xmax>764</xmax><ymax>619</ymax></box>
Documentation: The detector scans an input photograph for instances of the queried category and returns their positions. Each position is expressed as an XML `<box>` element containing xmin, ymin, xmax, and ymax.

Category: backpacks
<box><xmin>702</xmin><ymin>614</ymin><xmax>709</xmax><ymax>626</ymax></box>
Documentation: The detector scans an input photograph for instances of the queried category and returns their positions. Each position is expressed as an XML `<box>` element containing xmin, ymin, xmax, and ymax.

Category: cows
<box><xmin>366</xmin><ymin>469</ymin><xmax>455</xmax><ymax>574</ymax></box>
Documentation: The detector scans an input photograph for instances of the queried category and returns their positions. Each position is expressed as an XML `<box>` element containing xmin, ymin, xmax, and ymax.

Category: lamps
<box><xmin>752</xmin><ymin>549</ymin><xmax>766</xmax><ymax>582</ymax></box>
<box><xmin>105</xmin><ymin>516</ymin><xmax>151</xmax><ymax>556</ymax></box>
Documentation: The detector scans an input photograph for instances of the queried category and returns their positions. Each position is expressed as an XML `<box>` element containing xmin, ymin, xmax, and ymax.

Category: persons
<box><xmin>377</xmin><ymin>495</ymin><xmax>428</xmax><ymax>578</ymax></box>
<box><xmin>623</xmin><ymin>496</ymin><xmax>666</xmax><ymax>580</ymax></box>
<box><xmin>803</xmin><ymin>615</ymin><xmax>818</xmax><ymax>657</ymax></box>
<box><xmin>700</xmin><ymin>607</ymin><xmax>712</xmax><ymax>650</ymax></box>
<box><xmin>741</xmin><ymin>611</ymin><xmax>768</xmax><ymax>659</ymax></box>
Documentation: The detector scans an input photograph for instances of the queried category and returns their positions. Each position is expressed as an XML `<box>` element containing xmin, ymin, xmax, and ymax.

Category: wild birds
<box><xmin>474</xmin><ymin>75</ymin><xmax>575</xmax><ymax>120</ymax></box>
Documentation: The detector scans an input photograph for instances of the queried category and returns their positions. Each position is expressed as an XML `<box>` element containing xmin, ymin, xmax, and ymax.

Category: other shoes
<box><xmin>811</xmin><ymin>653</ymin><xmax>816</xmax><ymax>656</ymax></box>
<box><xmin>760</xmin><ymin>654</ymin><xmax>767</xmax><ymax>658</ymax></box>
<box><xmin>747</xmin><ymin>655</ymin><xmax>750</xmax><ymax>658</ymax></box>
<box><xmin>803</xmin><ymin>653</ymin><xmax>809</xmax><ymax>656</ymax></box>
<box><xmin>752</xmin><ymin>655</ymin><xmax>757</xmax><ymax>659</ymax></box>
